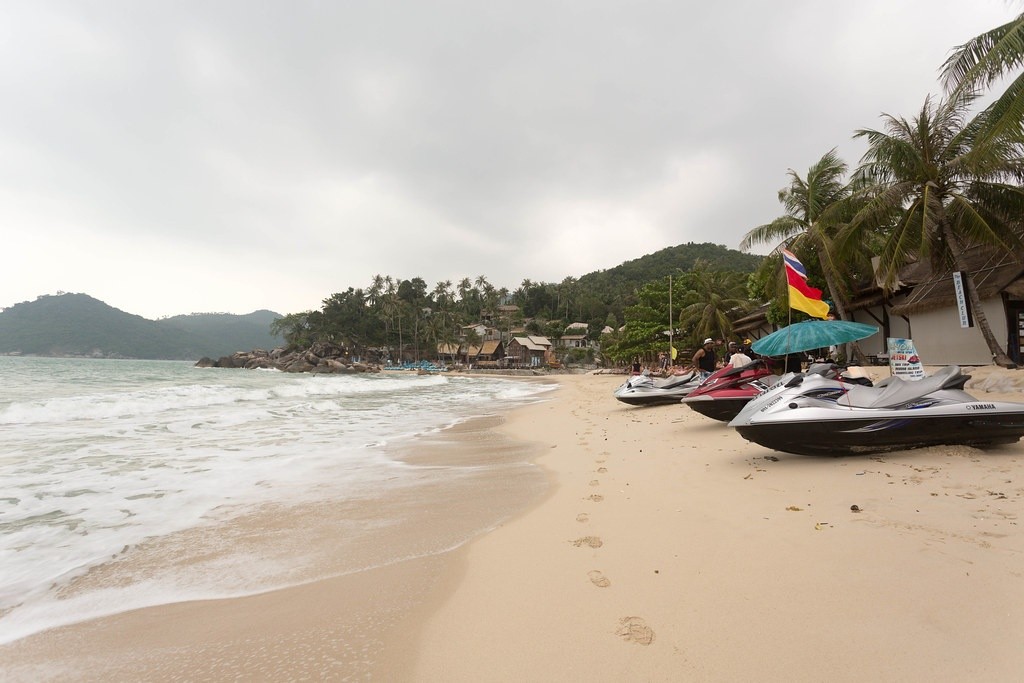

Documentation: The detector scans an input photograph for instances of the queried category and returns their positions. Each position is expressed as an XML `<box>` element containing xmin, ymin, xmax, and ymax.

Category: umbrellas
<box><xmin>750</xmin><ymin>317</ymin><xmax>879</xmax><ymax>356</ymax></box>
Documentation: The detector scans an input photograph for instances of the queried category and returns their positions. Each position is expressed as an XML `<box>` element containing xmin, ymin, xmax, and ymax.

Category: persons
<box><xmin>716</xmin><ymin>335</ymin><xmax>861</xmax><ymax>377</ymax></box>
<box><xmin>632</xmin><ymin>358</ymin><xmax>642</xmax><ymax>375</ymax></box>
<box><xmin>662</xmin><ymin>354</ymin><xmax>668</xmax><ymax>378</ymax></box>
<box><xmin>691</xmin><ymin>337</ymin><xmax>716</xmax><ymax>384</ymax></box>
<box><xmin>611</xmin><ymin>365</ymin><xmax>699</xmax><ymax>376</ymax></box>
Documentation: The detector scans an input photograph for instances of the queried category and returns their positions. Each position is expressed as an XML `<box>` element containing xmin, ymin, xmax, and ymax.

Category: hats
<box><xmin>743</xmin><ymin>339</ymin><xmax>753</xmax><ymax>345</ymax></box>
<box><xmin>703</xmin><ymin>338</ymin><xmax>715</xmax><ymax>344</ymax></box>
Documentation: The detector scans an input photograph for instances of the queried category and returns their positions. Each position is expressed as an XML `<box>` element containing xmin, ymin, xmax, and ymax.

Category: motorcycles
<box><xmin>612</xmin><ymin>365</ymin><xmax>712</xmax><ymax>406</ymax></box>
<box><xmin>727</xmin><ymin>362</ymin><xmax>1024</xmax><ymax>457</ymax></box>
<box><xmin>680</xmin><ymin>355</ymin><xmax>785</xmax><ymax>423</ymax></box>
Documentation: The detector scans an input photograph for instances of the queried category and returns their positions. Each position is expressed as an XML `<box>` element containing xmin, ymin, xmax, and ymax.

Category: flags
<box><xmin>783</xmin><ymin>247</ymin><xmax>834</xmax><ymax>320</ymax></box>
